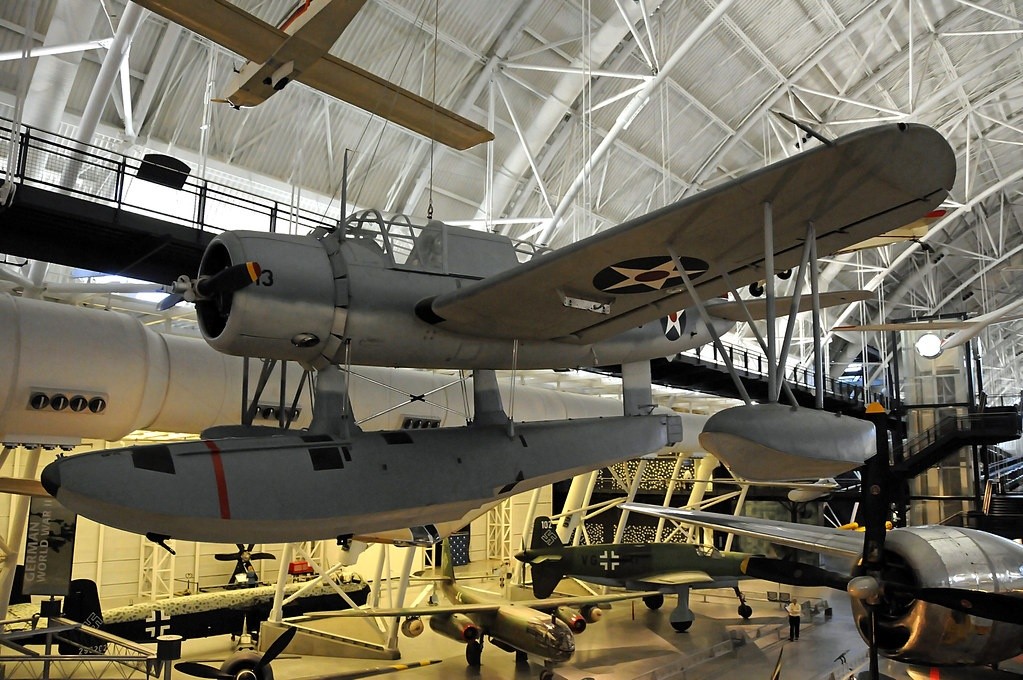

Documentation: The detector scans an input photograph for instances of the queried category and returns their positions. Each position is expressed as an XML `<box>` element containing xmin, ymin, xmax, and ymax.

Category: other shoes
<box><xmin>794</xmin><ymin>638</ymin><xmax>798</xmax><ymax>640</ymax></box>
<box><xmin>790</xmin><ymin>638</ymin><xmax>794</xmax><ymax>642</ymax></box>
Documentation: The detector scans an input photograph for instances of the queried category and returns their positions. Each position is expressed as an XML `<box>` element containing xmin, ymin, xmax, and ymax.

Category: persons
<box><xmin>246</xmin><ymin>566</ymin><xmax>255</xmax><ymax>588</ymax></box>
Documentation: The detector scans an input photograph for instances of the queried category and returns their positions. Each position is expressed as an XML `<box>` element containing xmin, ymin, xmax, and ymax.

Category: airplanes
<box><xmin>123</xmin><ymin>0</ymin><xmax>495</xmax><ymax>153</ymax></box>
<box><xmin>1</xmin><ymin>124</ymin><xmax>1023</xmax><ymax>680</ymax></box>
